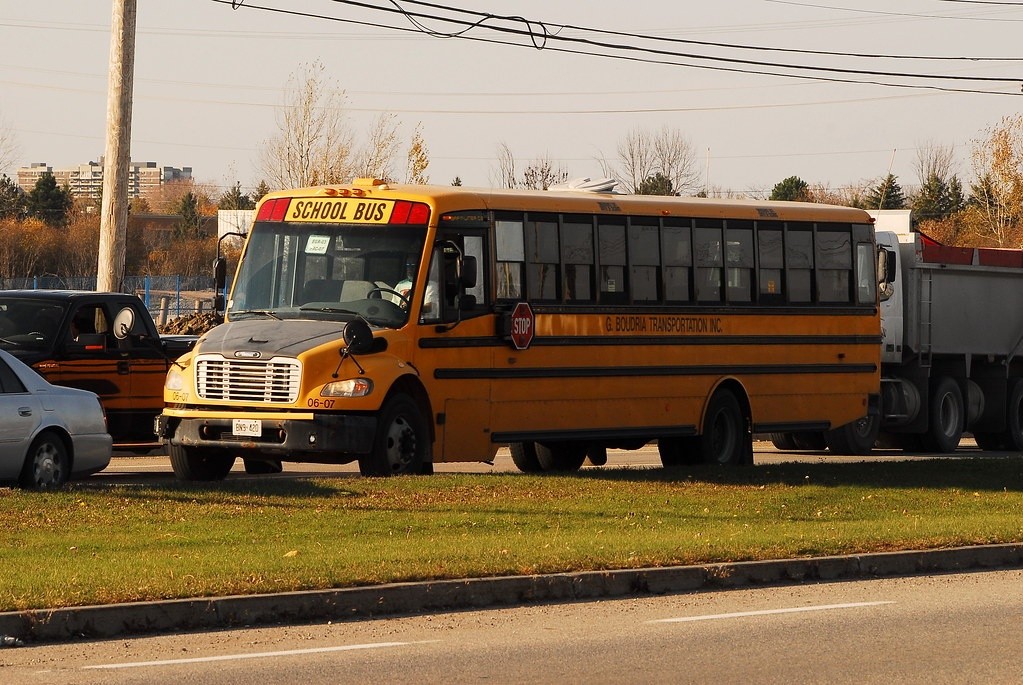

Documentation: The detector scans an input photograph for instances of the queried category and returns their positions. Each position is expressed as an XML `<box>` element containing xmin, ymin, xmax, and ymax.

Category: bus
<box><xmin>152</xmin><ymin>176</ymin><xmax>892</xmax><ymax>482</ymax></box>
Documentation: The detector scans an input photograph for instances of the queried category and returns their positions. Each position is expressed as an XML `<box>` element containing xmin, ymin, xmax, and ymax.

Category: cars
<box><xmin>0</xmin><ymin>349</ymin><xmax>114</xmax><ymax>487</ymax></box>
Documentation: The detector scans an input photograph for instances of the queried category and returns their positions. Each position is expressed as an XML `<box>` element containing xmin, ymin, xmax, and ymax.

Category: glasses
<box><xmin>405</xmin><ymin>264</ymin><xmax>417</xmax><ymax>268</ymax></box>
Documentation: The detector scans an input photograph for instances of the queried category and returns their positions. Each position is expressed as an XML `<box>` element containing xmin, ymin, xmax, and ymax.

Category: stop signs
<box><xmin>509</xmin><ymin>300</ymin><xmax>536</xmax><ymax>352</ymax></box>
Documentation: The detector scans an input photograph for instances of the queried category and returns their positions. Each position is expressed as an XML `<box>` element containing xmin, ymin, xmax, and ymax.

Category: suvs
<box><xmin>0</xmin><ymin>288</ymin><xmax>210</xmax><ymax>457</ymax></box>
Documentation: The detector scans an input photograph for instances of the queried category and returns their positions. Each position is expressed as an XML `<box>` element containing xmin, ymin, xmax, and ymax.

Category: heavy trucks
<box><xmin>768</xmin><ymin>209</ymin><xmax>1023</xmax><ymax>461</ymax></box>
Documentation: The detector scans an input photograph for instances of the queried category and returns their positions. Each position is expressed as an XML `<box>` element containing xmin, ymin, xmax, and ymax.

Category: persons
<box><xmin>72</xmin><ymin>314</ymin><xmax>82</xmax><ymax>339</ymax></box>
<box><xmin>390</xmin><ymin>254</ymin><xmax>439</xmax><ymax>319</ymax></box>
